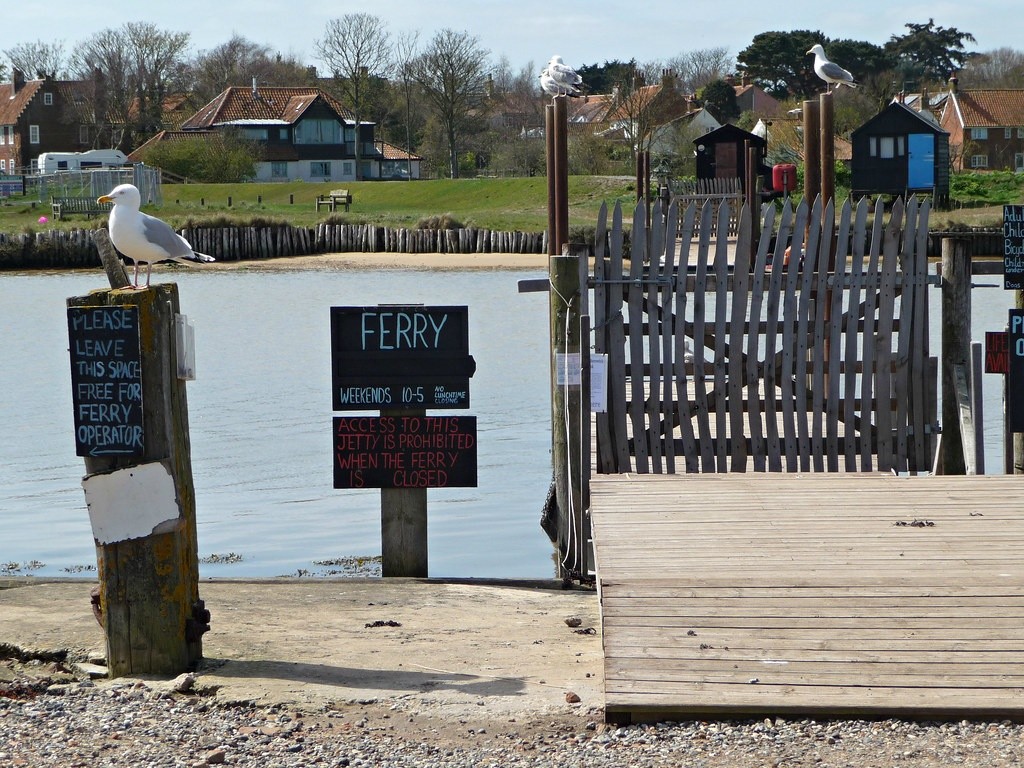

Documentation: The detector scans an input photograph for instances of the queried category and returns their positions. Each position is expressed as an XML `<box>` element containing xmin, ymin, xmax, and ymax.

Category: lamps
<box><xmin>698</xmin><ymin>145</ymin><xmax>706</xmax><ymax>155</ymax></box>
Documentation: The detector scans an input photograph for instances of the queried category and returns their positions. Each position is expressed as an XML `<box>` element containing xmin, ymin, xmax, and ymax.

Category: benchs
<box><xmin>51</xmin><ymin>196</ymin><xmax>115</xmax><ymax>221</ymax></box>
<box><xmin>316</xmin><ymin>189</ymin><xmax>352</xmax><ymax>213</ymax></box>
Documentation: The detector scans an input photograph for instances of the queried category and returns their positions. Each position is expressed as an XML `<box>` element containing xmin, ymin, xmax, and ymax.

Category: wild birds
<box><xmin>95</xmin><ymin>183</ymin><xmax>216</xmax><ymax>291</ymax></box>
<box><xmin>805</xmin><ymin>44</ymin><xmax>867</xmax><ymax>96</ymax></box>
